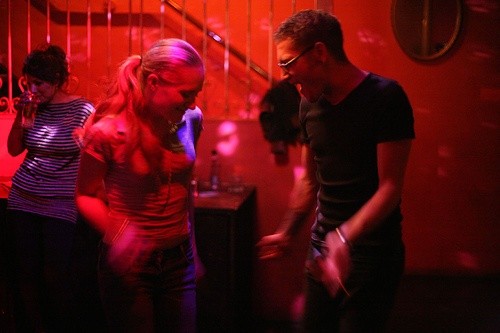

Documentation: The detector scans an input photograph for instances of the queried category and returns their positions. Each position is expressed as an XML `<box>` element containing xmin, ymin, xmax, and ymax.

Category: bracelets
<box><xmin>102</xmin><ymin>218</ymin><xmax>130</xmax><ymax>249</ymax></box>
<box><xmin>336</xmin><ymin>227</ymin><xmax>356</xmax><ymax>258</ymax></box>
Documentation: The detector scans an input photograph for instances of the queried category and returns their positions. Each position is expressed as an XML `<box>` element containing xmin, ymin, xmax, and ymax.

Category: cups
<box><xmin>19</xmin><ymin>90</ymin><xmax>39</xmax><ymax>129</ymax></box>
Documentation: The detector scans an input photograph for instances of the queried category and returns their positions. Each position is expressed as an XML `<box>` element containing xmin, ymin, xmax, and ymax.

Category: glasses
<box><xmin>277</xmin><ymin>45</ymin><xmax>314</xmax><ymax>73</ymax></box>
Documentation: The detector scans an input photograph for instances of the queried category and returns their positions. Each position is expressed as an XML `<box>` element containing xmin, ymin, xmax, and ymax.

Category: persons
<box><xmin>254</xmin><ymin>10</ymin><xmax>415</xmax><ymax>333</ymax></box>
<box><xmin>1</xmin><ymin>46</ymin><xmax>96</xmax><ymax>333</ymax></box>
<box><xmin>75</xmin><ymin>38</ymin><xmax>204</xmax><ymax>332</ymax></box>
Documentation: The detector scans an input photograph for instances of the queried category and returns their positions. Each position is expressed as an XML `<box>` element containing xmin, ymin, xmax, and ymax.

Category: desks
<box><xmin>193</xmin><ymin>185</ymin><xmax>257</xmax><ymax>278</ymax></box>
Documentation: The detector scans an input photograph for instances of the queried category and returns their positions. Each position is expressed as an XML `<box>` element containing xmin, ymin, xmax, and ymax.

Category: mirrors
<box><xmin>390</xmin><ymin>0</ymin><xmax>463</xmax><ymax>61</ymax></box>
